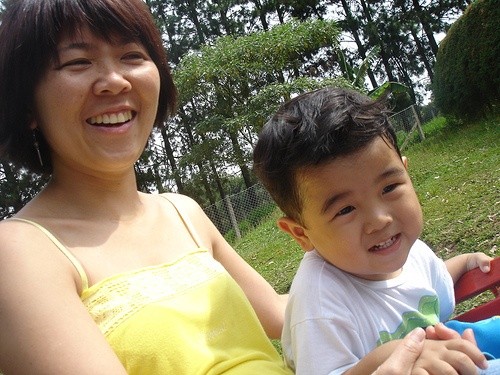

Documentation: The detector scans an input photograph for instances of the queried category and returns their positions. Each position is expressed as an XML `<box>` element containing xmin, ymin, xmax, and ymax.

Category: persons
<box><xmin>252</xmin><ymin>85</ymin><xmax>500</xmax><ymax>375</ymax></box>
<box><xmin>0</xmin><ymin>1</ymin><xmax>479</xmax><ymax>375</ymax></box>
